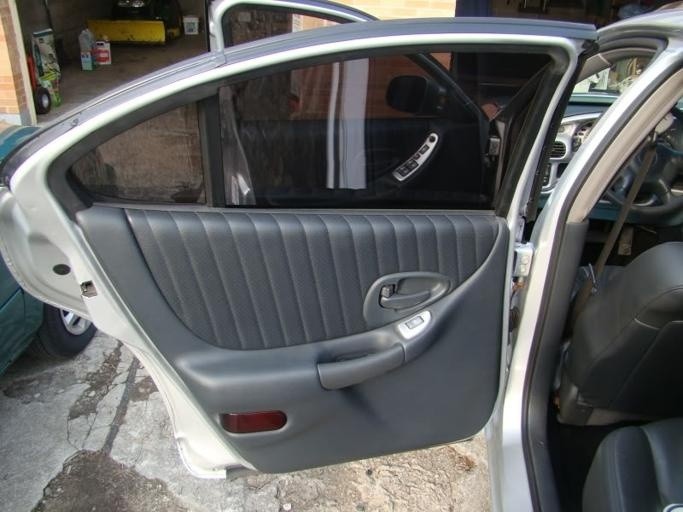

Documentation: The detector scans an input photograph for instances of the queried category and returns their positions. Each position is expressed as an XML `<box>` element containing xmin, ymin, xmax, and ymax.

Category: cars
<box><xmin>0</xmin><ymin>124</ymin><xmax>122</xmax><ymax>395</ymax></box>
<box><xmin>1</xmin><ymin>3</ymin><xmax>683</xmax><ymax>509</ymax></box>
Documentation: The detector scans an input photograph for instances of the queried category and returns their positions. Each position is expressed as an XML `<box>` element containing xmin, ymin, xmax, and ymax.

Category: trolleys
<box><xmin>25</xmin><ymin>53</ymin><xmax>51</xmax><ymax>114</ymax></box>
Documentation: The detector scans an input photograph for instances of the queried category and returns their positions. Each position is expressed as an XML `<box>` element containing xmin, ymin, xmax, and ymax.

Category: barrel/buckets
<box><xmin>94</xmin><ymin>40</ymin><xmax>111</xmax><ymax>66</ymax></box>
<box><xmin>80</xmin><ymin>50</ymin><xmax>93</xmax><ymax>71</ymax></box>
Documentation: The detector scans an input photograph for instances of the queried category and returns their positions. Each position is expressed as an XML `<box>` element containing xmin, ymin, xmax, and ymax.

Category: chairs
<box><xmin>582</xmin><ymin>416</ymin><xmax>683</xmax><ymax>511</ymax></box>
<box><xmin>553</xmin><ymin>241</ymin><xmax>683</xmax><ymax>456</ymax></box>
